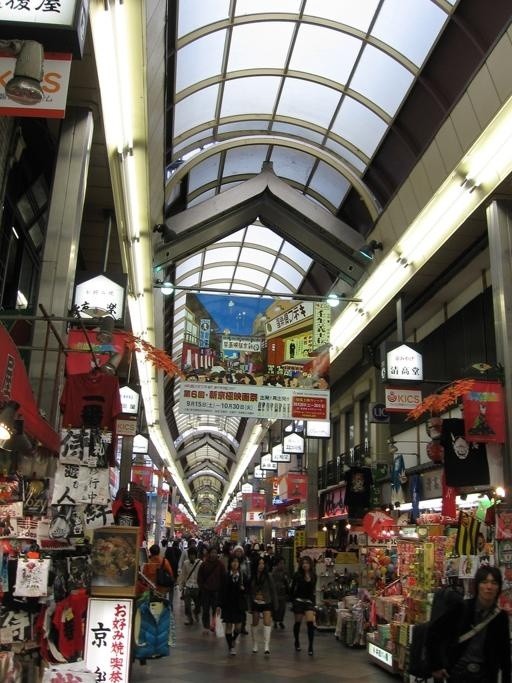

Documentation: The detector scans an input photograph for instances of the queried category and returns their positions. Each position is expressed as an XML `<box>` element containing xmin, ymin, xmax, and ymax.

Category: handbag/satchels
<box><xmin>156</xmin><ymin>568</ymin><xmax>175</xmax><ymax>588</ymax></box>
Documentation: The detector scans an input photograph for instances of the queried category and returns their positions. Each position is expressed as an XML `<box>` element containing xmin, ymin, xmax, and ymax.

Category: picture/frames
<box><xmin>90</xmin><ymin>526</ymin><xmax>140</xmax><ymax>596</ymax></box>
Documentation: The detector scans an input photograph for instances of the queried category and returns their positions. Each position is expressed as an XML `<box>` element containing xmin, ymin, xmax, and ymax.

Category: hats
<box><xmin>234</xmin><ymin>546</ymin><xmax>244</xmax><ymax>551</ymax></box>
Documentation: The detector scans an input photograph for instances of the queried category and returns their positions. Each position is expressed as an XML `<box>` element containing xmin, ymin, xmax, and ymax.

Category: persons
<box><xmin>426</xmin><ymin>565</ymin><xmax>512</xmax><ymax>683</ymax></box>
<box><xmin>142</xmin><ymin>527</ymin><xmax>318</xmax><ymax>656</ymax></box>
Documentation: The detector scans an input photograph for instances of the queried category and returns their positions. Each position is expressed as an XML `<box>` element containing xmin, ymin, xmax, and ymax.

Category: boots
<box><xmin>251</xmin><ymin>625</ymin><xmax>258</xmax><ymax>653</ymax></box>
<box><xmin>306</xmin><ymin>621</ymin><xmax>314</xmax><ymax>656</ymax></box>
<box><xmin>294</xmin><ymin>622</ymin><xmax>302</xmax><ymax>651</ymax></box>
<box><xmin>262</xmin><ymin>626</ymin><xmax>271</xmax><ymax>655</ymax></box>
<box><xmin>225</xmin><ymin>630</ymin><xmax>241</xmax><ymax>655</ymax></box>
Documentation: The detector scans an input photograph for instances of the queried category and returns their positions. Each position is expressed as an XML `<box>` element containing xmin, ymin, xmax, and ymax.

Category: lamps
<box><xmin>152</xmin><ymin>223</ymin><xmax>178</xmax><ymax>245</ymax></box>
<box><xmin>359</xmin><ymin>240</ymin><xmax>384</xmax><ymax>260</ymax></box>
<box><xmin>97</xmin><ymin>316</ymin><xmax>122</xmax><ymax>375</ymax></box>
<box><xmin>0</xmin><ymin>399</ymin><xmax>20</xmax><ymax>441</ymax></box>
